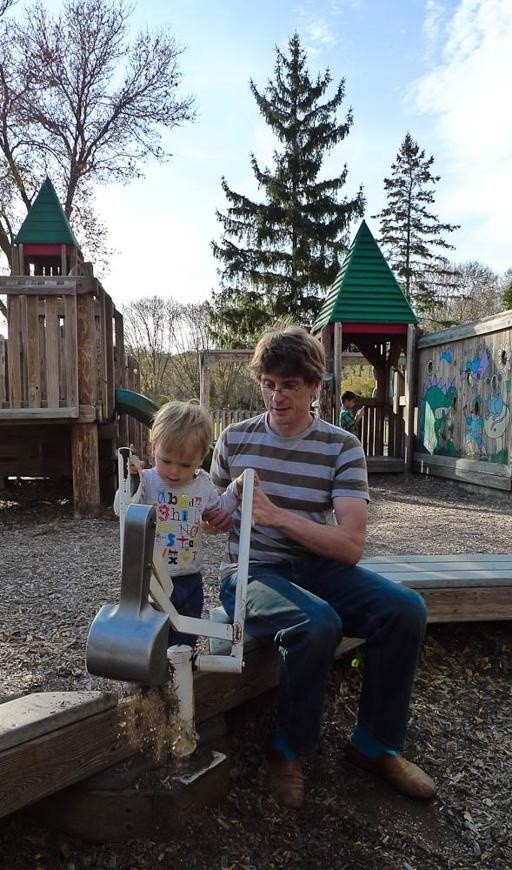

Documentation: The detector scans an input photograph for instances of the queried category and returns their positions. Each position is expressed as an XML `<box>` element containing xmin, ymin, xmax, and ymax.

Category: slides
<box><xmin>117</xmin><ymin>387</ymin><xmax>215</xmax><ymax>473</ymax></box>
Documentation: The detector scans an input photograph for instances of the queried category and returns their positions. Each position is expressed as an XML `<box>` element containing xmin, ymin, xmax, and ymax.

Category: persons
<box><xmin>340</xmin><ymin>390</ymin><xmax>366</xmax><ymax>431</ymax></box>
<box><xmin>201</xmin><ymin>326</ymin><xmax>437</xmax><ymax>810</ymax></box>
<box><xmin>113</xmin><ymin>397</ymin><xmax>259</xmax><ymax>657</ymax></box>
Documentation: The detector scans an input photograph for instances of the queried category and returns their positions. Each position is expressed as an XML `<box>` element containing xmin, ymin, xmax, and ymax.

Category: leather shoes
<box><xmin>265</xmin><ymin>744</ymin><xmax>306</xmax><ymax>809</ymax></box>
<box><xmin>348</xmin><ymin>744</ymin><xmax>437</xmax><ymax>801</ymax></box>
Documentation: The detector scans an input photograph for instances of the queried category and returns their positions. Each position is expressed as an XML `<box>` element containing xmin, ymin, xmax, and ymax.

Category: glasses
<box><xmin>257</xmin><ymin>384</ymin><xmax>306</xmax><ymax>395</ymax></box>
